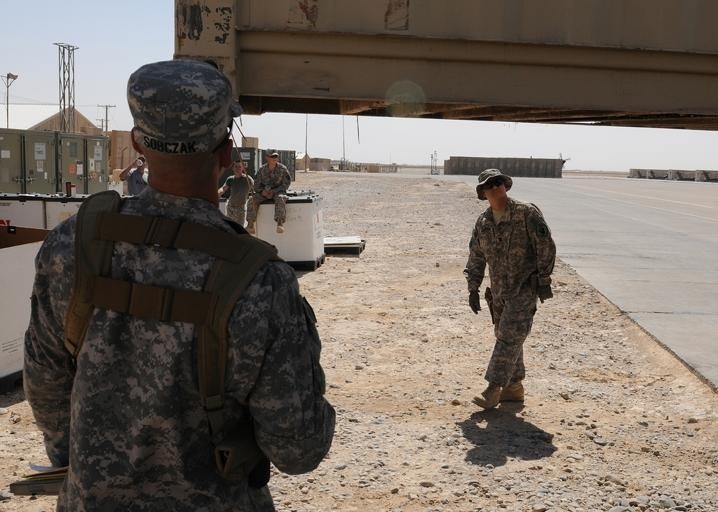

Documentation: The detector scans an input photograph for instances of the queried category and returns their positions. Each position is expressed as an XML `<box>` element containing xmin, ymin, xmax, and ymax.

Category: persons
<box><xmin>245</xmin><ymin>147</ymin><xmax>292</xmax><ymax>235</ymax></box>
<box><xmin>20</xmin><ymin>59</ymin><xmax>334</xmax><ymax>512</ymax></box>
<box><xmin>217</xmin><ymin>160</ymin><xmax>255</xmax><ymax>228</ymax></box>
<box><xmin>462</xmin><ymin>167</ymin><xmax>554</xmax><ymax>410</ymax></box>
<box><xmin>119</xmin><ymin>156</ymin><xmax>148</xmax><ymax>196</ymax></box>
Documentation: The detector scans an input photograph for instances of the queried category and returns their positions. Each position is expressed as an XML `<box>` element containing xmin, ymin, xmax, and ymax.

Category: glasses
<box><xmin>478</xmin><ymin>178</ymin><xmax>506</xmax><ymax>190</ymax></box>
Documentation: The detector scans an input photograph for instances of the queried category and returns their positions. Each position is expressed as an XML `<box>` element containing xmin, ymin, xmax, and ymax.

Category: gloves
<box><xmin>538</xmin><ymin>285</ymin><xmax>553</xmax><ymax>303</ymax></box>
<box><xmin>468</xmin><ymin>290</ymin><xmax>482</xmax><ymax>315</ymax></box>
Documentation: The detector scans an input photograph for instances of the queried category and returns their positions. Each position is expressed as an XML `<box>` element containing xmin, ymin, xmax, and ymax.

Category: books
<box><xmin>9</xmin><ymin>463</ymin><xmax>71</xmax><ymax>499</ymax></box>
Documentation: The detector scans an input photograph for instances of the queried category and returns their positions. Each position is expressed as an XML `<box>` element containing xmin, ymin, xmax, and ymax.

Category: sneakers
<box><xmin>244</xmin><ymin>226</ymin><xmax>256</xmax><ymax>234</ymax></box>
<box><xmin>471</xmin><ymin>381</ymin><xmax>524</xmax><ymax>409</ymax></box>
<box><xmin>276</xmin><ymin>225</ymin><xmax>285</xmax><ymax>233</ymax></box>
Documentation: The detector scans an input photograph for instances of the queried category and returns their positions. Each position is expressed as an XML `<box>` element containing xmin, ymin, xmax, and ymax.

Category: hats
<box><xmin>476</xmin><ymin>168</ymin><xmax>513</xmax><ymax>201</ymax></box>
<box><xmin>126</xmin><ymin>60</ymin><xmax>243</xmax><ymax>156</ymax></box>
<box><xmin>265</xmin><ymin>149</ymin><xmax>279</xmax><ymax>156</ymax></box>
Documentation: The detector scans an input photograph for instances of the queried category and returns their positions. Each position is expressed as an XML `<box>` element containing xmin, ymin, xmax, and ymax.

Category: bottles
<box><xmin>66</xmin><ymin>182</ymin><xmax>76</xmax><ymax>198</ymax></box>
